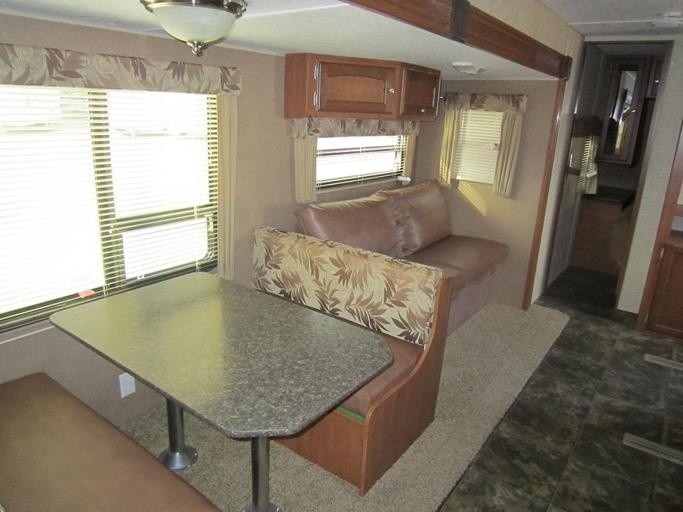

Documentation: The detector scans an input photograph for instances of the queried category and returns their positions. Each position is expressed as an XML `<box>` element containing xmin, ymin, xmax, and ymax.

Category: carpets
<box><xmin>116</xmin><ymin>297</ymin><xmax>570</xmax><ymax>512</ymax></box>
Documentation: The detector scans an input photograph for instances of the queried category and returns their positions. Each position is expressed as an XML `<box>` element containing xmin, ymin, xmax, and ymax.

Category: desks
<box><xmin>47</xmin><ymin>269</ymin><xmax>396</xmax><ymax>512</ymax></box>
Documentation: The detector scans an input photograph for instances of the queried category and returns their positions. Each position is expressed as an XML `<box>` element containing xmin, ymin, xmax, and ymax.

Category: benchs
<box><xmin>247</xmin><ymin>226</ymin><xmax>456</xmax><ymax>496</ymax></box>
<box><xmin>0</xmin><ymin>371</ymin><xmax>221</xmax><ymax>511</ymax></box>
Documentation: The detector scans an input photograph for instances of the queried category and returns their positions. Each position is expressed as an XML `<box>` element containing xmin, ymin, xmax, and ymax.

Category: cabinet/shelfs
<box><xmin>644</xmin><ymin>247</ymin><xmax>682</xmax><ymax>339</ymax></box>
<box><xmin>282</xmin><ymin>50</ymin><xmax>442</xmax><ymax>123</ymax></box>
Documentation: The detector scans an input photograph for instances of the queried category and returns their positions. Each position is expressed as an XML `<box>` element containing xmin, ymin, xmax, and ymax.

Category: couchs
<box><xmin>294</xmin><ymin>176</ymin><xmax>511</xmax><ymax>340</ymax></box>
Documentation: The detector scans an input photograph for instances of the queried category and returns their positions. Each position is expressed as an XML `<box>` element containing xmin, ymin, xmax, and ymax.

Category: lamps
<box><xmin>137</xmin><ymin>0</ymin><xmax>248</xmax><ymax>58</ymax></box>
